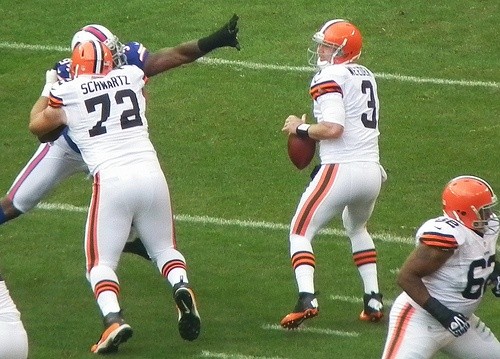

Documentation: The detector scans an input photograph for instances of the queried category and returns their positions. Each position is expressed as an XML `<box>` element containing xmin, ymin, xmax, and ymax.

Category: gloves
<box><xmin>422</xmin><ymin>296</ymin><xmax>470</xmax><ymax>337</ymax></box>
<box><xmin>487</xmin><ymin>270</ymin><xmax>500</xmax><ymax>297</ymax></box>
<box><xmin>39</xmin><ymin>124</ymin><xmax>66</xmax><ymax>143</ymax></box>
<box><xmin>198</xmin><ymin>14</ymin><xmax>241</xmax><ymax>54</ymax></box>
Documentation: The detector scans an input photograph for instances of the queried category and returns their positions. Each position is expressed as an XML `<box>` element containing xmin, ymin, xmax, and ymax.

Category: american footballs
<box><xmin>287</xmin><ymin>123</ymin><xmax>316</xmax><ymax>170</ymax></box>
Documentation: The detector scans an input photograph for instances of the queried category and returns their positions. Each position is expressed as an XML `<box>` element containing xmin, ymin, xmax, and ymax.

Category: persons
<box><xmin>0</xmin><ymin>272</ymin><xmax>29</xmax><ymax>359</ymax></box>
<box><xmin>0</xmin><ymin>14</ymin><xmax>241</xmax><ymax>261</ymax></box>
<box><xmin>279</xmin><ymin>19</ymin><xmax>387</xmax><ymax>330</ymax></box>
<box><xmin>29</xmin><ymin>40</ymin><xmax>202</xmax><ymax>355</ymax></box>
<box><xmin>381</xmin><ymin>175</ymin><xmax>500</xmax><ymax>359</ymax></box>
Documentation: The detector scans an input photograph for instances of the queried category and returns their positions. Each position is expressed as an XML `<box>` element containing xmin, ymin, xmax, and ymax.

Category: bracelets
<box><xmin>296</xmin><ymin>124</ymin><xmax>310</xmax><ymax>138</ymax></box>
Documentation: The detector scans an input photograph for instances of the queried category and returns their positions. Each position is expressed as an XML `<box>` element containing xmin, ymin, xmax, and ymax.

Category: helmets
<box><xmin>307</xmin><ymin>18</ymin><xmax>362</xmax><ymax>71</ymax></box>
<box><xmin>442</xmin><ymin>176</ymin><xmax>500</xmax><ymax>235</ymax></box>
<box><xmin>70</xmin><ymin>40</ymin><xmax>113</xmax><ymax>81</ymax></box>
<box><xmin>70</xmin><ymin>24</ymin><xmax>128</xmax><ymax>69</ymax></box>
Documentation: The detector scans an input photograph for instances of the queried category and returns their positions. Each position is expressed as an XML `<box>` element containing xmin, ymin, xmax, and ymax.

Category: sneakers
<box><xmin>172</xmin><ymin>282</ymin><xmax>201</xmax><ymax>341</ymax></box>
<box><xmin>360</xmin><ymin>291</ymin><xmax>384</xmax><ymax>322</ymax></box>
<box><xmin>91</xmin><ymin>312</ymin><xmax>132</xmax><ymax>354</ymax></box>
<box><xmin>280</xmin><ymin>291</ymin><xmax>319</xmax><ymax>330</ymax></box>
